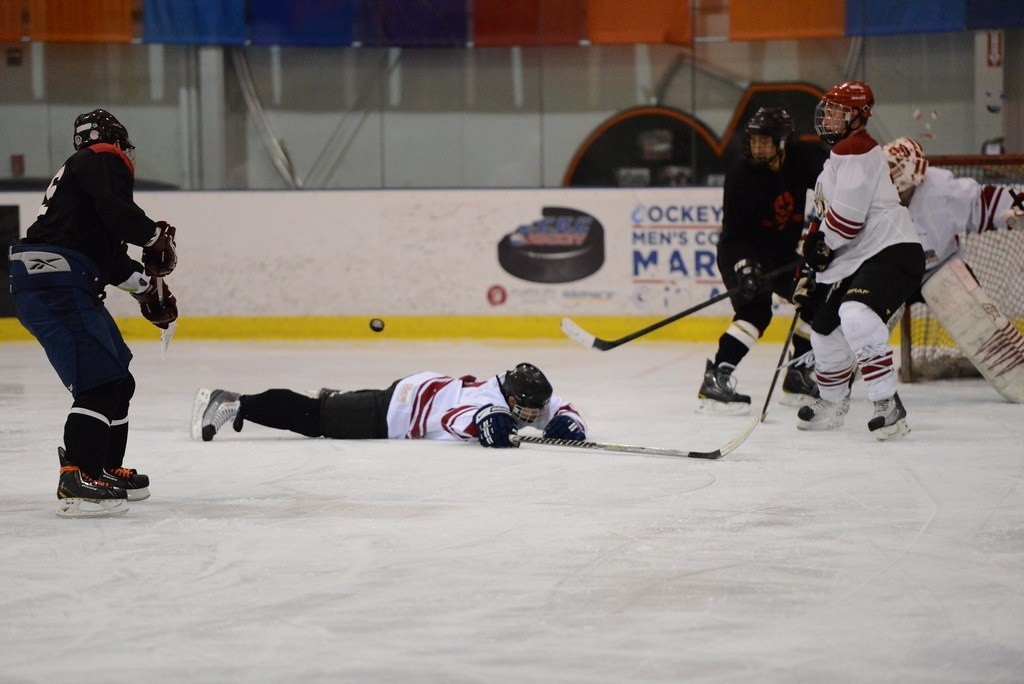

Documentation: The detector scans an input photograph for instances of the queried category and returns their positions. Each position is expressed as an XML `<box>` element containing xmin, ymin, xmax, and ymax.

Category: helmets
<box><xmin>73</xmin><ymin>108</ymin><xmax>135</xmax><ymax>151</ymax></box>
<box><xmin>501</xmin><ymin>362</ymin><xmax>553</xmax><ymax>428</ymax></box>
<box><xmin>882</xmin><ymin>136</ymin><xmax>928</xmax><ymax>191</ymax></box>
<box><xmin>812</xmin><ymin>81</ymin><xmax>874</xmax><ymax>145</ymax></box>
<box><xmin>740</xmin><ymin>107</ymin><xmax>796</xmax><ymax>171</ymax></box>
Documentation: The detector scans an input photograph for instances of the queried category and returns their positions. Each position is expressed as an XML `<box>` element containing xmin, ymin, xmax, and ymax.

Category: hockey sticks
<box><xmin>559</xmin><ymin>254</ymin><xmax>797</xmax><ymax>352</ymax></box>
<box><xmin>760</xmin><ymin>305</ymin><xmax>802</xmax><ymax>423</ymax></box>
<box><xmin>508</xmin><ymin>413</ymin><xmax>759</xmax><ymax>461</ymax></box>
<box><xmin>156</xmin><ymin>275</ymin><xmax>176</xmax><ymax>356</ymax></box>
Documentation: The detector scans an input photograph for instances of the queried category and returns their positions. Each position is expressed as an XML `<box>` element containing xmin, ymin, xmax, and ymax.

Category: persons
<box><xmin>799</xmin><ymin>81</ymin><xmax>914</xmax><ymax>441</ymax></box>
<box><xmin>807</xmin><ymin>137</ymin><xmax>1024</xmax><ymax>404</ymax></box>
<box><xmin>192</xmin><ymin>363</ymin><xmax>587</xmax><ymax>449</ymax></box>
<box><xmin>693</xmin><ymin>106</ymin><xmax>817</xmax><ymax>420</ymax></box>
<box><xmin>7</xmin><ymin>109</ymin><xmax>178</xmax><ymax>519</ymax></box>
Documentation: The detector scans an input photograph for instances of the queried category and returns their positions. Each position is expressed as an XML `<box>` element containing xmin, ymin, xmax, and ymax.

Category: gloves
<box><xmin>130</xmin><ymin>276</ymin><xmax>178</xmax><ymax>330</ymax></box>
<box><xmin>542</xmin><ymin>416</ymin><xmax>586</xmax><ymax>440</ymax></box>
<box><xmin>473</xmin><ymin>403</ymin><xmax>519</xmax><ymax>447</ymax></box>
<box><xmin>733</xmin><ymin>257</ymin><xmax>765</xmax><ymax>298</ymax></box>
<box><xmin>791</xmin><ymin>272</ymin><xmax>816</xmax><ymax>306</ymax></box>
<box><xmin>800</xmin><ymin>227</ymin><xmax>836</xmax><ymax>272</ymax></box>
<box><xmin>141</xmin><ymin>220</ymin><xmax>177</xmax><ymax>277</ymax></box>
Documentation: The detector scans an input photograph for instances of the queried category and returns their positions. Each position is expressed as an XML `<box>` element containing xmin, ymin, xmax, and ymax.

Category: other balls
<box><xmin>370</xmin><ymin>318</ymin><xmax>385</xmax><ymax>332</ymax></box>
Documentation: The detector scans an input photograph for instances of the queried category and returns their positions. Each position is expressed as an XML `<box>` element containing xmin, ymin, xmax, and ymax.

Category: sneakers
<box><xmin>189</xmin><ymin>388</ymin><xmax>243</xmax><ymax>442</ymax></box>
<box><xmin>796</xmin><ymin>369</ymin><xmax>856</xmax><ymax>431</ymax></box>
<box><xmin>54</xmin><ymin>447</ymin><xmax>130</xmax><ymax>517</ymax></box>
<box><xmin>100</xmin><ymin>466</ymin><xmax>151</xmax><ymax>500</ymax></box>
<box><xmin>776</xmin><ymin>351</ymin><xmax>821</xmax><ymax>406</ymax></box>
<box><xmin>867</xmin><ymin>390</ymin><xmax>912</xmax><ymax>440</ymax></box>
<box><xmin>694</xmin><ymin>359</ymin><xmax>752</xmax><ymax>415</ymax></box>
<box><xmin>304</xmin><ymin>387</ymin><xmax>351</xmax><ymax>397</ymax></box>
